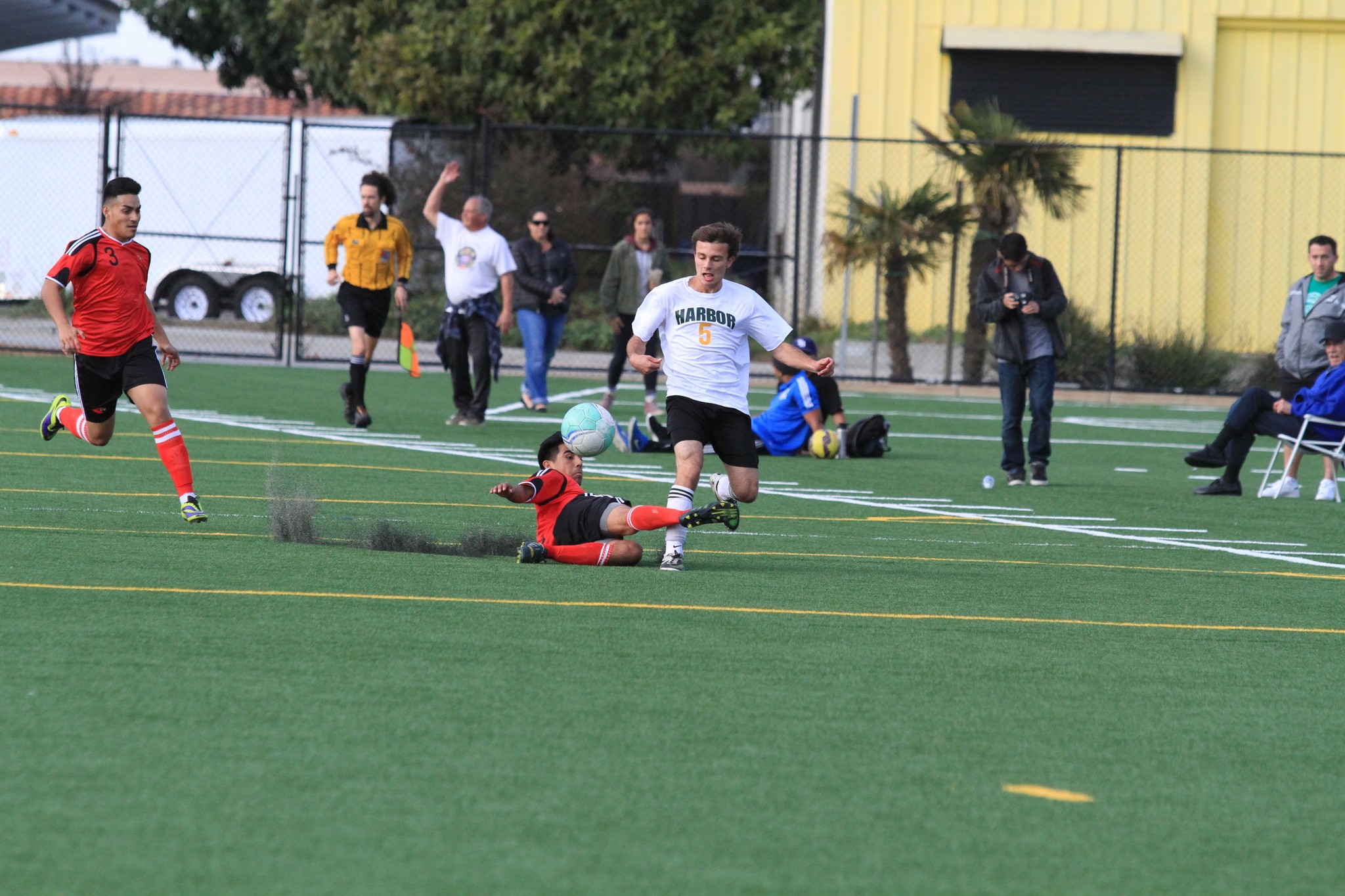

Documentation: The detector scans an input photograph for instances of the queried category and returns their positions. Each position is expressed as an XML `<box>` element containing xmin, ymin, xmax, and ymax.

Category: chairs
<box><xmin>1257</xmin><ymin>414</ymin><xmax>1345</xmax><ymax>503</ymax></box>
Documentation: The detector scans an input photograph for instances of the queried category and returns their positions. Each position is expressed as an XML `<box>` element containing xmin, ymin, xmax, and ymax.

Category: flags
<box><xmin>396</xmin><ymin>319</ymin><xmax>420</xmax><ymax>377</ymax></box>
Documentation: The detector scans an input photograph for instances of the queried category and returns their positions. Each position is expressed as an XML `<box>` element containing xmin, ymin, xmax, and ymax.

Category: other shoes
<box><xmin>534</xmin><ymin>403</ymin><xmax>546</xmax><ymax>412</ymax></box>
<box><xmin>445</xmin><ymin>412</ymin><xmax>465</xmax><ymax>425</ymax></box>
<box><xmin>521</xmin><ymin>394</ymin><xmax>534</xmax><ymax>412</ymax></box>
<box><xmin>459</xmin><ymin>416</ymin><xmax>485</xmax><ymax>427</ymax></box>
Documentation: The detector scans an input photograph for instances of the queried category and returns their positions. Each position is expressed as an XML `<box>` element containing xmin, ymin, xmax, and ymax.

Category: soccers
<box><xmin>809</xmin><ymin>429</ymin><xmax>839</xmax><ymax>460</ymax></box>
<box><xmin>560</xmin><ymin>403</ymin><xmax>617</xmax><ymax>457</ymax></box>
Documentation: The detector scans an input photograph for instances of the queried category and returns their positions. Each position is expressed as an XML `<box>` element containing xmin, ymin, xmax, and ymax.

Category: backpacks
<box><xmin>846</xmin><ymin>413</ymin><xmax>890</xmax><ymax>458</ymax></box>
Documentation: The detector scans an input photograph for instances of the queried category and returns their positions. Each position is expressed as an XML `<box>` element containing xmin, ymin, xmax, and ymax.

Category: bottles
<box><xmin>837</xmin><ymin>422</ymin><xmax>848</xmax><ymax>460</ymax></box>
<box><xmin>982</xmin><ymin>475</ymin><xmax>994</xmax><ymax>488</ymax></box>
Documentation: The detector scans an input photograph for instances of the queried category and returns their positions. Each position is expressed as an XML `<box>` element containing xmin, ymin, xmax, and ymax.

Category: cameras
<box><xmin>1012</xmin><ymin>292</ymin><xmax>1032</xmax><ymax>309</ymax></box>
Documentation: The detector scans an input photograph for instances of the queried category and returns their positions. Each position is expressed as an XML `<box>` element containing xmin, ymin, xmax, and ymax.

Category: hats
<box><xmin>792</xmin><ymin>338</ymin><xmax>819</xmax><ymax>356</ymax></box>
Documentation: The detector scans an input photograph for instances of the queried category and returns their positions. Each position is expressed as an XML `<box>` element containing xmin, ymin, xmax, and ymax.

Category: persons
<box><xmin>323</xmin><ymin>170</ymin><xmax>414</xmax><ymax>430</ymax></box>
<box><xmin>507</xmin><ymin>207</ymin><xmax>576</xmax><ymax>413</ymax></box>
<box><xmin>625</xmin><ymin>224</ymin><xmax>834</xmax><ymax>572</ymax></box>
<box><xmin>1184</xmin><ymin>233</ymin><xmax>1345</xmax><ymax>499</ymax></box>
<box><xmin>613</xmin><ymin>338</ymin><xmax>849</xmax><ymax>458</ymax></box>
<box><xmin>39</xmin><ymin>177</ymin><xmax>207</xmax><ymax>523</ymax></box>
<box><xmin>973</xmin><ymin>232</ymin><xmax>1067</xmax><ymax>486</ymax></box>
<box><xmin>599</xmin><ymin>209</ymin><xmax>673</xmax><ymax>419</ymax></box>
<box><xmin>422</xmin><ymin>160</ymin><xmax>520</xmax><ymax>426</ymax></box>
<box><xmin>488</xmin><ymin>430</ymin><xmax>741</xmax><ymax>566</ymax></box>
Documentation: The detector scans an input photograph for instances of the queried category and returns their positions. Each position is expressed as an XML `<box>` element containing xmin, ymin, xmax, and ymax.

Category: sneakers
<box><xmin>645</xmin><ymin>399</ymin><xmax>665</xmax><ymax>417</ymax></box>
<box><xmin>1194</xmin><ymin>478</ymin><xmax>1242</xmax><ymax>495</ymax></box>
<box><xmin>180</xmin><ymin>495</ymin><xmax>208</xmax><ymax>524</ymax></box>
<box><xmin>660</xmin><ymin>551</ymin><xmax>684</xmax><ymax>572</ymax></box>
<box><xmin>612</xmin><ymin>420</ymin><xmax>629</xmax><ymax>453</ymax></box>
<box><xmin>1259</xmin><ymin>476</ymin><xmax>1300</xmax><ymax>498</ymax></box>
<box><xmin>516</xmin><ymin>541</ymin><xmax>548</xmax><ymax>564</ymax></box>
<box><xmin>340</xmin><ymin>382</ymin><xmax>354</xmax><ymax>425</ymax></box>
<box><xmin>627</xmin><ymin>417</ymin><xmax>648</xmax><ymax>453</ymax></box>
<box><xmin>679</xmin><ymin>501</ymin><xmax>739</xmax><ymax>528</ymax></box>
<box><xmin>40</xmin><ymin>395</ymin><xmax>71</xmax><ymax>441</ymax></box>
<box><xmin>1006</xmin><ymin>468</ymin><xmax>1026</xmax><ymax>486</ymax></box>
<box><xmin>600</xmin><ymin>393</ymin><xmax>614</xmax><ymax>411</ymax></box>
<box><xmin>645</xmin><ymin>412</ymin><xmax>668</xmax><ymax>443</ymax></box>
<box><xmin>1030</xmin><ymin>463</ymin><xmax>1048</xmax><ymax>486</ymax></box>
<box><xmin>1315</xmin><ymin>479</ymin><xmax>1337</xmax><ymax>502</ymax></box>
<box><xmin>1184</xmin><ymin>444</ymin><xmax>1228</xmax><ymax>469</ymax></box>
<box><xmin>710</xmin><ymin>472</ymin><xmax>740</xmax><ymax>532</ymax></box>
<box><xmin>353</xmin><ymin>405</ymin><xmax>372</xmax><ymax>428</ymax></box>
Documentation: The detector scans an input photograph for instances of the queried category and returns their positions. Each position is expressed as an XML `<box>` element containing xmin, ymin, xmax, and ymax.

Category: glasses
<box><xmin>531</xmin><ymin>219</ymin><xmax>550</xmax><ymax>226</ymax></box>
